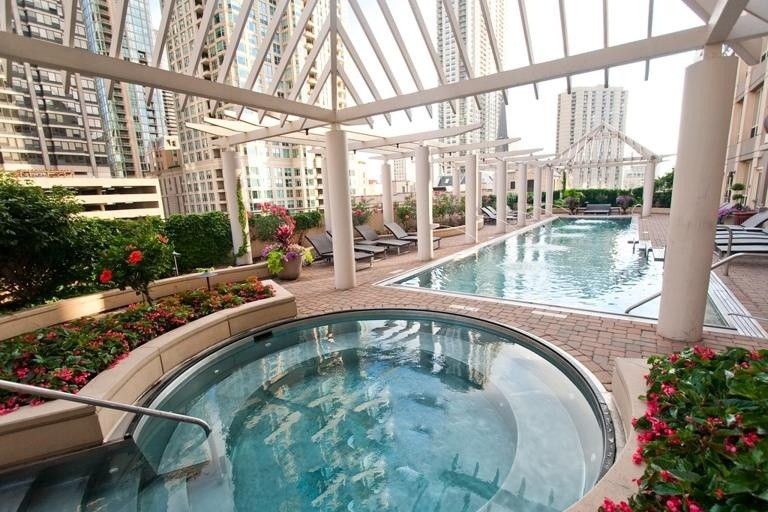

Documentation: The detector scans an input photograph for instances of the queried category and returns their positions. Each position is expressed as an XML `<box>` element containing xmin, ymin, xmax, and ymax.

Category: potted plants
<box><xmin>730</xmin><ymin>182</ymin><xmax>757</xmax><ymax>225</ymax></box>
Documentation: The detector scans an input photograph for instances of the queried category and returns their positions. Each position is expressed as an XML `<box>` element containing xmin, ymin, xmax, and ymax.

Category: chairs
<box><xmin>305</xmin><ymin>224</ymin><xmax>443</xmax><ymax>268</ymax></box>
<box><xmin>480</xmin><ymin>204</ymin><xmax>531</xmax><ymax>226</ymax></box>
<box><xmin>714</xmin><ymin>202</ymin><xmax>768</xmax><ymax>260</ymax></box>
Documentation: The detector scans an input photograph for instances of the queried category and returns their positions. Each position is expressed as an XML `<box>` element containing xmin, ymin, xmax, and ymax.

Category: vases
<box><xmin>569</xmin><ymin>208</ymin><xmax>575</xmax><ymax>215</ymax></box>
<box><xmin>275</xmin><ymin>255</ymin><xmax>303</xmax><ymax>280</ymax></box>
<box><xmin>622</xmin><ymin>207</ymin><xmax>629</xmax><ymax>215</ymax></box>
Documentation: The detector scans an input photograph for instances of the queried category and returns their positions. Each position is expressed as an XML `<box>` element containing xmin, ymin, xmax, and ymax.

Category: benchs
<box><xmin>584</xmin><ymin>203</ymin><xmax>612</xmax><ymax>216</ymax></box>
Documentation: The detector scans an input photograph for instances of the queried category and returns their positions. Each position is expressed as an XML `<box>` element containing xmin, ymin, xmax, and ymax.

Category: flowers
<box><xmin>565</xmin><ymin>197</ymin><xmax>578</xmax><ymax>208</ymax></box>
<box><xmin>260</xmin><ymin>224</ymin><xmax>315</xmax><ymax>276</ymax></box>
<box><xmin>617</xmin><ymin>195</ymin><xmax>634</xmax><ymax>208</ymax></box>
